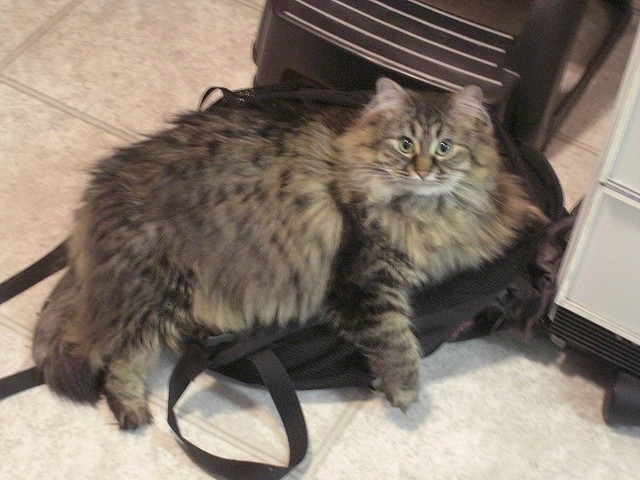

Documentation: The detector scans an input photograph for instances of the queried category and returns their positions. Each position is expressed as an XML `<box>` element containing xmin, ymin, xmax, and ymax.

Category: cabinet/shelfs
<box><xmin>542</xmin><ymin>21</ymin><xmax>640</xmax><ymax>427</ymax></box>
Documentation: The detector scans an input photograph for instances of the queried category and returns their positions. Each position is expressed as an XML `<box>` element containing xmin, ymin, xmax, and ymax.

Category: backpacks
<box><xmin>0</xmin><ymin>85</ymin><xmax>565</xmax><ymax>479</ymax></box>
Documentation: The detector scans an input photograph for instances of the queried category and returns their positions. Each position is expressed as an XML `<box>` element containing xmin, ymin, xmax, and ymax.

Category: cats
<box><xmin>31</xmin><ymin>77</ymin><xmax>552</xmax><ymax>431</ymax></box>
